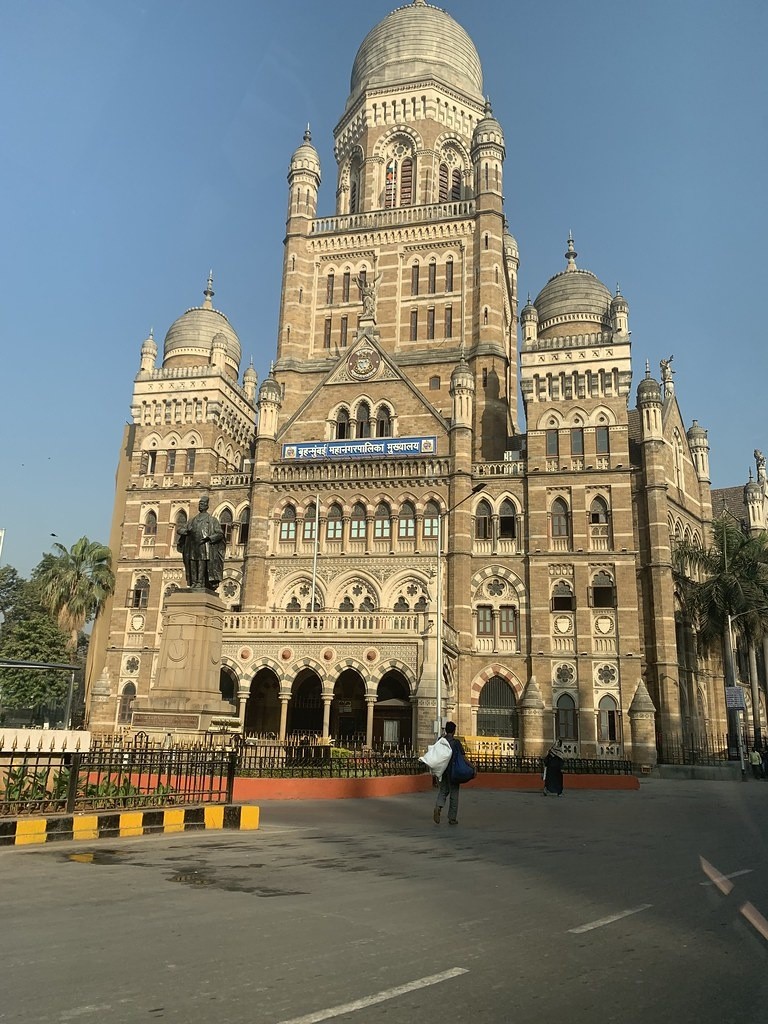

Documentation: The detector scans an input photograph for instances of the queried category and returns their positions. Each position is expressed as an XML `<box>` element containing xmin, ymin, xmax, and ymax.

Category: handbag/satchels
<box><xmin>446</xmin><ymin>739</ymin><xmax>478</xmax><ymax>784</ymax></box>
<box><xmin>541</xmin><ymin>766</ymin><xmax>546</xmax><ymax>780</ymax></box>
<box><xmin>419</xmin><ymin>736</ymin><xmax>453</xmax><ymax>779</ymax></box>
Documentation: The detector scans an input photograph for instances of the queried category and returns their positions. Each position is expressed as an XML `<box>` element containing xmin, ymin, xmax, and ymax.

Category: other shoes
<box><xmin>448</xmin><ymin>819</ymin><xmax>458</xmax><ymax>825</ymax></box>
<box><xmin>558</xmin><ymin>792</ymin><xmax>564</xmax><ymax>797</ymax></box>
<box><xmin>542</xmin><ymin>788</ymin><xmax>548</xmax><ymax>796</ymax></box>
<box><xmin>433</xmin><ymin>807</ymin><xmax>441</xmax><ymax>824</ymax></box>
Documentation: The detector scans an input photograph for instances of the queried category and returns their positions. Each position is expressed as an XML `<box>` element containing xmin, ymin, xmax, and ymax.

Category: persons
<box><xmin>542</xmin><ymin>739</ymin><xmax>564</xmax><ymax>797</ymax></box>
<box><xmin>749</xmin><ymin>747</ymin><xmax>768</xmax><ymax>779</ymax></box>
<box><xmin>177</xmin><ymin>496</ymin><xmax>226</xmax><ymax>590</ymax></box>
<box><xmin>433</xmin><ymin>721</ymin><xmax>465</xmax><ymax>825</ymax></box>
